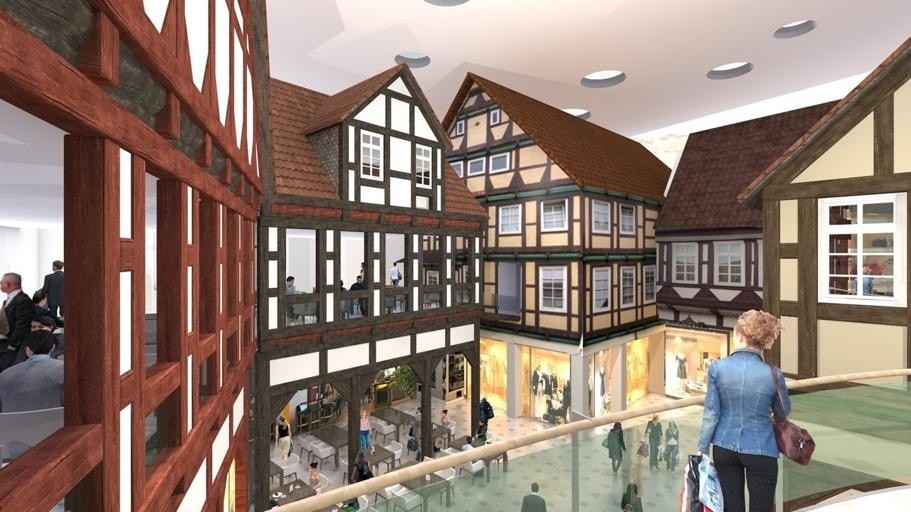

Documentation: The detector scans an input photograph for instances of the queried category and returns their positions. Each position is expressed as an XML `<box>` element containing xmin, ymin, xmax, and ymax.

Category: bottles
<box><xmin>426</xmin><ymin>474</ymin><xmax>431</xmax><ymax>480</ymax></box>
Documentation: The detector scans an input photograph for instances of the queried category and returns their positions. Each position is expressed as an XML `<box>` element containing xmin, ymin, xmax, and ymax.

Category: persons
<box><xmin>697</xmin><ymin>308</ymin><xmax>792</xmax><ymax>512</ymax></box>
<box><xmin>360</xmin><ymin>409</ymin><xmax>372</xmax><ymax>449</ymax></box>
<box><xmin>606</xmin><ymin>415</ymin><xmax>679</xmax><ymax>511</ymax></box>
<box><xmin>339</xmin><ymin>263</ymin><xmax>364</xmax><ymax>291</ymax></box>
<box><xmin>351</xmin><ymin>451</ymin><xmax>371</xmax><ymax>482</ymax></box>
<box><xmin>532</xmin><ymin>369</ymin><xmax>541</xmax><ymax>395</ymax></box>
<box><xmin>308</xmin><ymin>462</ymin><xmax>322</xmax><ymax>490</ymax></box>
<box><xmin>475</xmin><ymin>417</ymin><xmax>487</xmax><ymax>441</ymax></box>
<box><xmin>441</xmin><ymin>410</ymin><xmax>450</xmax><ymax>429</ymax></box>
<box><xmin>389</xmin><ymin>262</ymin><xmax>401</xmax><ymax>286</ymax></box>
<box><xmin>481</xmin><ymin>398</ymin><xmax>492</xmax><ymax>430</ymax></box>
<box><xmin>675</xmin><ymin>352</ymin><xmax>688</xmax><ymax>380</ymax></box>
<box><xmin>853</xmin><ymin>267</ymin><xmax>873</xmax><ymax>293</ymax></box>
<box><xmin>275</xmin><ymin>415</ymin><xmax>292</xmax><ymax>460</ymax></box>
<box><xmin>413</xmin><ymin>407</ymin><xmax>422</xmax><ymax>461</ymax></box>
<box><xmin>286</xmin><ymin>276</ymin><xmax>299</xmax><ymax>294</ymax></box>
<box><xmin>599</xmin><ymin>367</ymin><xmax>605</xmax><ymax>397</ymax></box>
<box><xmin>521</xmin><ymin>483</ymin><xmax>546</xmax><ymax>512</ymax></box>
<box><xmin>0</xmin><ymin>261</ymin><xmax>66</xmax><ymax>412</ymax></box>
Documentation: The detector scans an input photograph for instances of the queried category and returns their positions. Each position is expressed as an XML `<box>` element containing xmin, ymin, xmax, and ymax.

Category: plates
<box><xmin>272</xmin><ymin>491</ymin><xmax>287</xmax><ymax>501</ymax></box>
